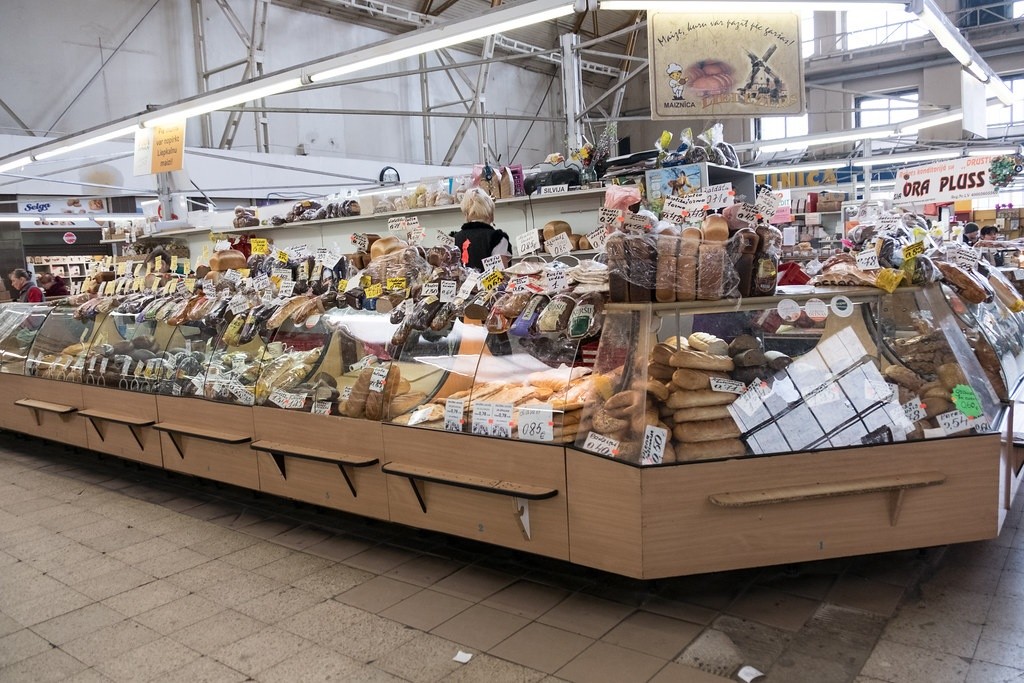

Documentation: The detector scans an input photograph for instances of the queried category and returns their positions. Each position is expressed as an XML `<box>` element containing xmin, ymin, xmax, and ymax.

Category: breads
<box><xmin>583</xmin><ymin>215</ymin><xmax>1024</xmax><ymax>466</ymax></box>
<box><xmin>30</xmin><ymin>187</ymin><xmax>626</xmax><ymax>443</ymax></box>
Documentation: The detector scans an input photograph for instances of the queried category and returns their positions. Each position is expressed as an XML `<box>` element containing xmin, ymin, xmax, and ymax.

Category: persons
<box><xmin>451</xmin><ymin>189</ymin><xmax>512</xmax><ymax>273</ymax></box>
<box><xmin>146</xmin><ymin>247</ymin><xmax>172</xmax><ymax>273</ymax></box>
<box><xmin>41</xmin><ymin>273</ymin><xmax>69</xmax><ymax>296</ymax></box>
<box><xmin>963</xmin><ymin>223</ymin><xmax>979</xmax><ymax>246</ymax></box>
<box><xmin>974</xmin><ymin>226</ymin><xmax>1003</xmax><ymax>276</ymax></box>
<box><xmin>10</xmin><ymin>269</ymin><xmax>46</xmax><ymax>330</ymax></box>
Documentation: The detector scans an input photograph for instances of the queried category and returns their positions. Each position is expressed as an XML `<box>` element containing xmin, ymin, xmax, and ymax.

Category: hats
<box><xmin>980</xmin><ymin>226</ymin><xmax>998</xmax><ymax>235</ymax></box>
<box><xmin>963</xmin><ymin>222</ymin><xmax>979</xmax><ymax>234</ymax></box>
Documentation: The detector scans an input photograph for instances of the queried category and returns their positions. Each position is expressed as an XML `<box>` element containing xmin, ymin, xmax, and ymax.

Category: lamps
<box><xmin>588</xmin><ymin>0</ymin><xmax>1015</xmax><ymax>174</ymax></box>
<box><xmin>0</xmin><ymin>0</ymin><xmax>586</xmax><ymax>173</ymax></box>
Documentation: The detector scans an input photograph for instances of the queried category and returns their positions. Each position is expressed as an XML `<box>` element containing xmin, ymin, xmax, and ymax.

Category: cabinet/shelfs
<box><xmin>99</xmin><ymin>186</ymin><xmax>606</xmax><ymax>262</ymax></box>
